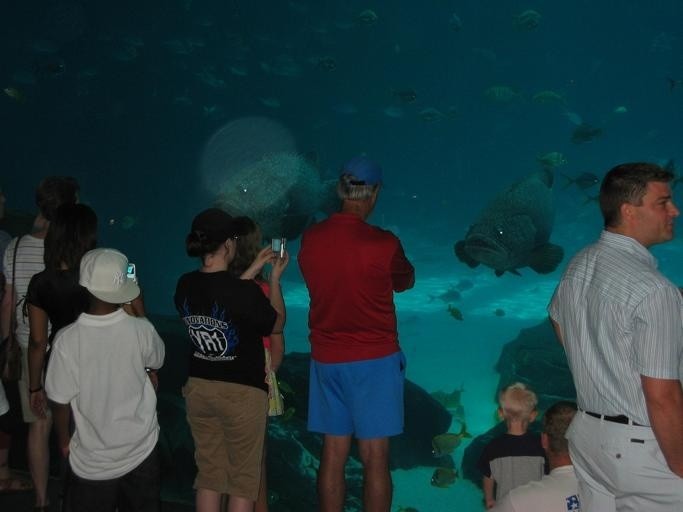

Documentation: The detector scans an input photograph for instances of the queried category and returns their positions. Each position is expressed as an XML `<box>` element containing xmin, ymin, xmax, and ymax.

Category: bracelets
<box><xmin>28</xmin><ymin>385</ymin><xmax>44</xmax><ymax>393</ymax></box>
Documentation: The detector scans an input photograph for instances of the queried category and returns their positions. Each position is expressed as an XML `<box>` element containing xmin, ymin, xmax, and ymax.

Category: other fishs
<box><xmin>0</xmin><ymin>0</ymin><xmax>683</xmax><ymax>322</ymax></box>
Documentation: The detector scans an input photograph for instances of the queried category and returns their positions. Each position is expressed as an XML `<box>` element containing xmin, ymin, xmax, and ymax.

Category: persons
<box><xmin>482</xmin><ymin>400</ymin><xmax>585</xmax><ymax>511</ymax></box>
<box><xmin>545</xmin><ymin>161</ymin><xmax>682</xmax><ymax>511</ymax></box>
<box><xmin>0</xmin><ymin>179</ymin><xmax>39</xmax><ymax>491</ymax></box>
<box><xmin>295</xmin><ymin>152</ymin><xmax>415</xmax><ymax>511</ymax></box>
<box><xmin>169</xmin><ymin>206</ymin><xmax>289</xmax><ymax>512</ymax></box>
<box><xmin>478</xmin><ymin>381</ymin><xmax>551</xmax><ymax>511</ymax></box>
<box><xmin>19</xmin><ymin>201</ymin><xmax>111</xmax><ymax>510</ymax></box>
<box><xmin>229</xmin><ymin>216</ymin><xmax>284</xmax><ymax>511</ymax></box>
<box><xmin>0</xmin><ymin>174</ymin><xmax>83</xmax><ymax>511</ymax></box>
<box><xmin>42</xmin><ymin>246</ymin><xmax>166</xmax><ymax>510</ymax></box>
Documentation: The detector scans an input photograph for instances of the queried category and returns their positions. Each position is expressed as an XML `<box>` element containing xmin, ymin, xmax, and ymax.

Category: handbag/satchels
<box><xmin>0</xmin><ymin>334</ymin><xmax>22</xmax><ymax>382</ymax></box>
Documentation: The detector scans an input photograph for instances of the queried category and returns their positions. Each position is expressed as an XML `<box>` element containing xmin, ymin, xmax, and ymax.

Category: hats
<box><xmin>77</xmin><ymin>248</ymin><xmax>140</xmax><ymax>305</ymax></box>
<box><xmin>339</xmin><ymin>156</ymin><xmax>383</xmax><ymax>187</ymax></box>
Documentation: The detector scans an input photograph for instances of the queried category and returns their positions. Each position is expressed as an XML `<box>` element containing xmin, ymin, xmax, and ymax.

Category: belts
<box><xmin>579</xmin><ymin>408</ymin><xmax>643</xmax><ymax>427</ymax></box>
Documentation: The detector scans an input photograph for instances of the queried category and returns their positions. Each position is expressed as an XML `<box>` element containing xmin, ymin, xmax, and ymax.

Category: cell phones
<box><xmin>271</xmin><ymin>233</ymin><xmax>286</xmax><ymax>266</ymax></box>
<box><xmin>127</xmin><ymin>263</ymin><xmax>137</xmax><ymax>285</ymax></box>
